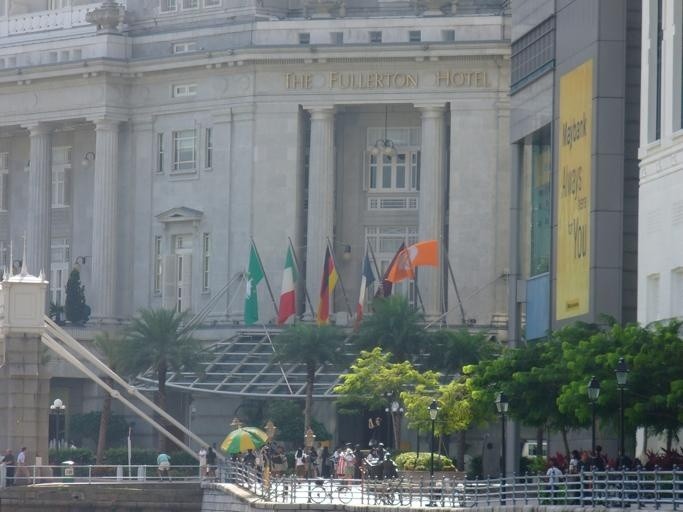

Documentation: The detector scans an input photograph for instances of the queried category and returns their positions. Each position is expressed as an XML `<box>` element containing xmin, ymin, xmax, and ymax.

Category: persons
<box><xmin>0</xmin><ymin>447</ymin><xmax>13</xmax><ymax>486</ymax></box>
<box><xmin>545</xmin><ymin>445</ymin><xmax>605</xmax><ymax>505</ymax></box>
<box><xmin>14</xmin><ymin>446</ymin><xmax>30</xmax><ymax>484</ymax></box>
<box><xmin>156</xmin><ymin>452</ymin><xmax>175</xmax><ymax>480</ymax></box>
<box><xmin>198</xmin><ymin>439</ymin><xmax>397</xmax><ymax>492</ymax></box>
<box><xmin>463</xmin><ymin>442</ymin><xmax>477</xmax><ymax>472</ymax></box>
<box><xmin>482</xmin><ymin>442</ymin><xmax>499</xmax><ymax>473</ymax></box>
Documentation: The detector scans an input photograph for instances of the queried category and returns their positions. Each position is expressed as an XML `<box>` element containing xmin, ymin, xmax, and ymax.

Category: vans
<box><xmin>520</xmin><ymin>439</ymin><xmax>547</xmax><ymax>482</ymax></box>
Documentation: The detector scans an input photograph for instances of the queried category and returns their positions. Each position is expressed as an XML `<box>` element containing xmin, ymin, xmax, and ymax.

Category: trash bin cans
<box><xmin>60</xmin><ymin>460</ymin><xmax>77</xmax><ymax>483</ymax></box>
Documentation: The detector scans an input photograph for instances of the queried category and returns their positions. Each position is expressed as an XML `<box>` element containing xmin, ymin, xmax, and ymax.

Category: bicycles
<box><xmin>310</xmin><ymin>474</ymin><xmax>353</xmax><ymax>504</ymax></box>
<box><xmin>598</xmin><ymin>483</ymin><xmax>662</xmax><ymax>508</ymax></box>
<box><xmin>375</xmin><ymin>489</ymin><xmax>413</xmax><ymax>506</ymax></box>
<box><xmin>458</xmin><ymin>490</ymin><xmax>476</xmax><ymax>507</ymax></box>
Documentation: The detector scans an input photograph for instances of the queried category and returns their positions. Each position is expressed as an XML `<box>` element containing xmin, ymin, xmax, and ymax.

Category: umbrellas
<box><xmin>220</xmin><ymin>426</ymin><xmax>267</xmax><ymax>454</ymax></box>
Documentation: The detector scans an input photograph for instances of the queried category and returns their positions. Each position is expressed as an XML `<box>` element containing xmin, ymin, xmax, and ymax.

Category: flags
<box><xmin>243</xmin><ymin>234</ymin><xmax>439</xmax><ymax>334</ymax></box>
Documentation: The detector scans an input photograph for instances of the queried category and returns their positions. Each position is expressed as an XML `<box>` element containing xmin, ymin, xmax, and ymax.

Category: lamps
<box><xmin>363</xmin><ymin>104</ymin><xmax>398</xmax><ymax>162</ymax></box>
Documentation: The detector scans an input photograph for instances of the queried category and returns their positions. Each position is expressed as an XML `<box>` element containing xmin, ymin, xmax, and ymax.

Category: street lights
<box><xmin>425</xmin><ymin>399</ymin><xmax>440</xmax><ymax>507</ymax></box>
<box><xmin>588</xmin><ymin>376</ymin><xmax>600</xmax><ymax>469</ymax></box>
<box><xmin>264</xmin><ymin>419</ymin><xmax>277</xmax><ymax>502</ymax></box>
<box><xmin>384</xmin><ymin>401</ymin><xmax>403</xmax><ymax>450</ymax></box>
<box><xmin>495</xmin><ymin>391</ymin><xmax>509</xmax><ymax>505</ymax></box>
<box><xmin>230</xmin><ymin>416</ymin><xmax>242</xmax><ymax>482</ymax></box>
<box><xmin>613</xmin><ymin>357</ymin><xmax>630</xmax><ymax>508</ymax></box>
<box><xmin>304</xmin><ymin>426</ymin><xmax>317</xmax><ymax>503</ymax></box>
<box><xmin>51</xmin><ymin>398</ymin><xmax>66</xmax><ymax>475</ymax></box>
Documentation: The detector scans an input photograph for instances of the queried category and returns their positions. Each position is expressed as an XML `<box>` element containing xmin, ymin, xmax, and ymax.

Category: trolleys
<box><xmin>363</xmin><ymin>457</ymin><xmax>399</xmax><ymax>491</ymax></box>
